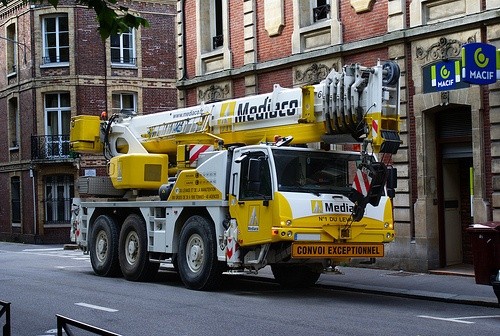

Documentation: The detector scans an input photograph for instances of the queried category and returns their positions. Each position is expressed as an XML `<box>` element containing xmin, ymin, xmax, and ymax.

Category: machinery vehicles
<box><xmin>66</xmin><ymin>60</ymin><xmax>404</xmax><ymax>286</ymax></box>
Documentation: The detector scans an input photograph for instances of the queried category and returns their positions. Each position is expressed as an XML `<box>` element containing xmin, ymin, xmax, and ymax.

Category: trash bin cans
<box><xmin>466</xmin><ymin>222</ymin><xmax>499</xmax><ymax>285</ymax></box>
